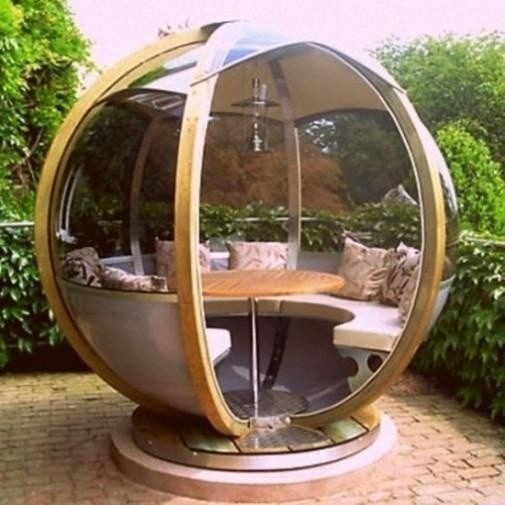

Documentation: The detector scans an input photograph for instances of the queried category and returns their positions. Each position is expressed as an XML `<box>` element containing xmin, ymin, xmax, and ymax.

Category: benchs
<box><xmin>54</xmin><ymin>252</ymin><xmax>457</xmax><ymax>392</ymax></box>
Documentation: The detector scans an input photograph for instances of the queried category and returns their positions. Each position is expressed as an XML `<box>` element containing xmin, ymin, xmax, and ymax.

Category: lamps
<box><xmin>231</xmin><ymin>61</ymin><xmax>280</xmax><ymax>156</ymax></box>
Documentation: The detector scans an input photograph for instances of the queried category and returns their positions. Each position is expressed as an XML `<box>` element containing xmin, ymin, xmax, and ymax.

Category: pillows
<box><xmin>378</xmin><ymin>242</ymin><xmax>420</xmax><ymax>327</ymax></box>
<box><xmin>331</xmin><ymin>235</ymin><xmax>392</xmax><ymax>305</ymax></box>
<box><xmin>224</xmin><ymin>240</ymin><xmax>290</xmax><ymax>270</ymax></box>
<box><xmin>154</xmin><ymin>237</ymin><xmax>213</xmax><ymax>279</ymax></box>
<box><xmin>58</xmin><ymin>247</ymin><xmax>168</xmax><ymax>293</ymax></box>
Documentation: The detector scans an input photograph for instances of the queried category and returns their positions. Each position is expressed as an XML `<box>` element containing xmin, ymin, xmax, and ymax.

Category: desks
<box><xmin>164</xmin><ymin>268</ymin><xmax>347</xmax><ymax>422</ymax></box>
